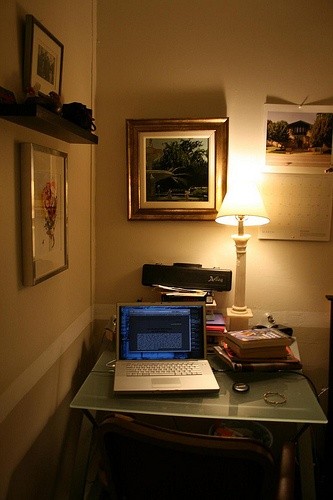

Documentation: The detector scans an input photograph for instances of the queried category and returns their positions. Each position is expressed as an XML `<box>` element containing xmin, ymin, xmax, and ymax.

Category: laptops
<box><xmin>114</xmin><ymin>302</ymin><xmax>220</xmax><ymax>395</ymax></box>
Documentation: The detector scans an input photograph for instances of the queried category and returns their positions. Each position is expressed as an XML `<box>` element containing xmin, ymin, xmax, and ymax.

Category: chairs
<box><xmin>95</xmin><ymin>413</ymin><xmax>294</xmax><ymax>500</ymax></box>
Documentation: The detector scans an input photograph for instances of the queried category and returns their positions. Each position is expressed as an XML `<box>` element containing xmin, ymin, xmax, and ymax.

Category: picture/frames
<box><xmin>21</xmin><ymin>13</ymin><xmax>65</xmax><ymax>97</ymax></box>
<box><xmin>123</xmin><ymin>116</ymin><xmax>230</xmax><ymax>221</ymax></box>
<box><xmin>20</xmin><ymin>140</ymin><xmax>70</xmax><ymax>287</ymax></box>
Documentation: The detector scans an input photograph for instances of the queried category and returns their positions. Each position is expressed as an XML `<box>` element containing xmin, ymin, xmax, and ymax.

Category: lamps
<box><xmin>215</xmin><ymin>176</ymin><xmax>270</xmax><ymax>331</ymax></box>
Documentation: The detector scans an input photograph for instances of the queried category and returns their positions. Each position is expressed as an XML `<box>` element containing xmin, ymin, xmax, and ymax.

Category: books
<box><xmin>153</xmin><ymin>284</ymin><xmax>226</xmax><ymax>355</ymax></box>
<box><xmin>211</xmin><ymin>327</ymin><xmax>303</xmax><ymax>377</ymax></box>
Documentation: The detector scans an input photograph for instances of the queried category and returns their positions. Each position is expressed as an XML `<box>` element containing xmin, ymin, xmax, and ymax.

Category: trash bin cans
<box><xmin>209</xmin><ymin>420</ymin><xmax>272</xmax><ymax>449</ymax></box>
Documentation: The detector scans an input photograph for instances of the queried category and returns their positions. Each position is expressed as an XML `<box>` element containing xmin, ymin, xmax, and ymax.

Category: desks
<box><xmin>69</xmin><ymin>338</ymin><xmax>329</xmax><ymax>439</ymax></box>
